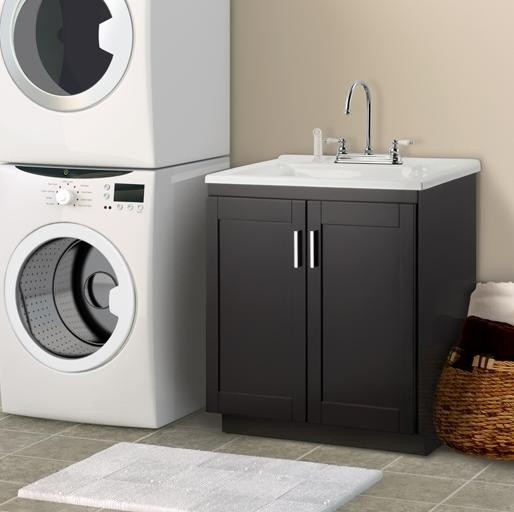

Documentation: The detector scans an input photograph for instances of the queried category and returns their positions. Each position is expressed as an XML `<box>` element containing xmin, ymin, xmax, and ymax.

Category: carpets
<box><xmin>18</xmin><ymin>440</ymin><xmax>382</xmax><ymax>512</ymax></box>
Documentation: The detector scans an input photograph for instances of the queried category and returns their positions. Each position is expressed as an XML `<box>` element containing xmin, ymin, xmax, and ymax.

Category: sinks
<box><xmin>205</xmin><ymin>153</ymin><xmax>481</xmax><ymax>191</ymax></box>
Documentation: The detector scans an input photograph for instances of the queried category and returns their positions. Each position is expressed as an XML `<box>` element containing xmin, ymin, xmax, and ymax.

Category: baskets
<box><xmin>430</xmin><ymin>347</ymin><xmax>514</xmax><ymax>463</ymax></box>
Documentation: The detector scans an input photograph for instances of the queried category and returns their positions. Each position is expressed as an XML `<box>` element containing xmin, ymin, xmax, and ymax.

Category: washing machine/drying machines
<box><xmin>0</xmin><ymin>164</ymin><xmax>230</xmax><ymax>429</ymax></box>
<box><xmin>0</xmin><ymin>0</ymin><xmax>231</xmax><ymax>169</ymax></box>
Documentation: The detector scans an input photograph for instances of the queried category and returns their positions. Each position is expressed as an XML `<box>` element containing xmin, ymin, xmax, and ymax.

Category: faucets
<box><xmin>343</xmin><ymin>81</ymin><xmax>371</xmax><ymax>147</ymax></box>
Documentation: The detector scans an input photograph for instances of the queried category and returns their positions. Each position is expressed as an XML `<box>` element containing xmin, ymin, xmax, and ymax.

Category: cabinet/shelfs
<box><xmin>205</xmin><ymin>174</ymin><xmax>479</xmax><ymax>455</ymax></box>
